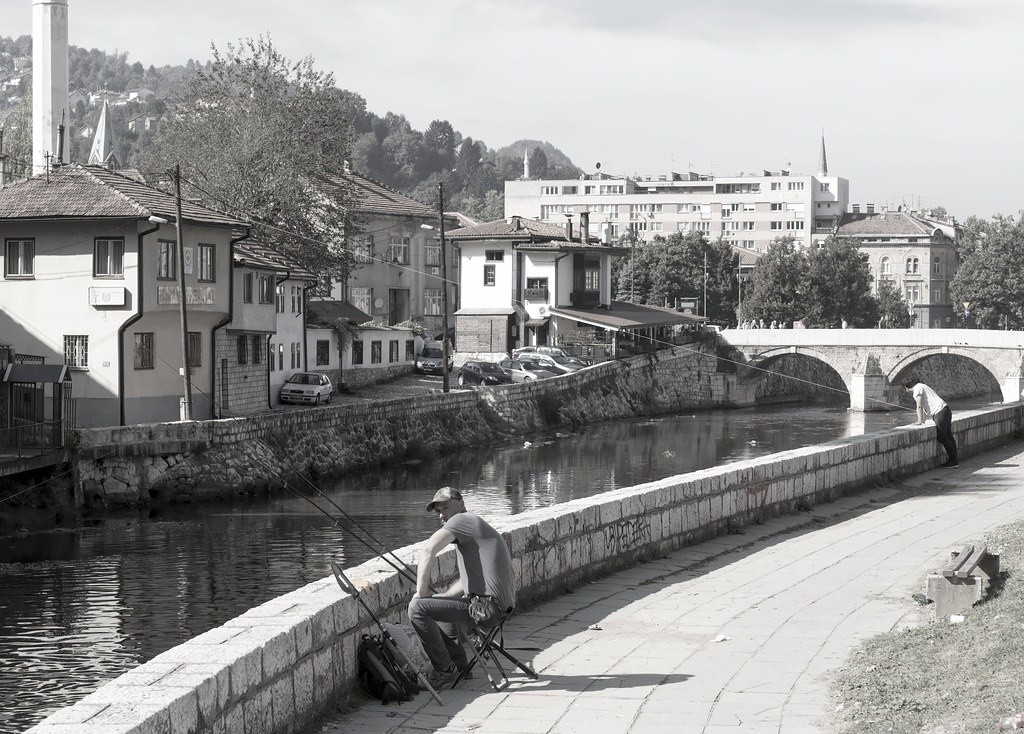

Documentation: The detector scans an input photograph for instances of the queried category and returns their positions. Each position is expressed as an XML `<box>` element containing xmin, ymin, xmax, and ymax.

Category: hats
<box><xmin>426</xmin><ymin>486</ymin><xmax>462</xmax><ymax>512</ymax></box>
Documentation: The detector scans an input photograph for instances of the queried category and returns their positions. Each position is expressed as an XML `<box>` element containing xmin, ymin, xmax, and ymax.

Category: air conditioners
<box><xmin>538</xmin><ymin>305</ymin><xmax>552</xmax><ymax>317</ymax></box>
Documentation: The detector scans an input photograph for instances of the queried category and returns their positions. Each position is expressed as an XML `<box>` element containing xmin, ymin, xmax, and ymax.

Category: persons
<box><xmin>903</xmin><ymin>376</ymin><xmax>960</xmax><ymax>468</ymax></box>
<box><xmin>743</xmin><ymin>316</ymin><xmax>886</xmax><ymax>329</ymax></box>
<box><xmin>408</xmin><ymin>487</ymin><xmax>516</xmax><ymax>690</ymax></box>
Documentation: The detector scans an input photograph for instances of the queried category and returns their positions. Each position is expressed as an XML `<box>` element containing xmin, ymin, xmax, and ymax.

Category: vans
<box><xmin>416</xmin><ymin>340</ymin><xmax>455</xmax><ymax>374</ymax></box>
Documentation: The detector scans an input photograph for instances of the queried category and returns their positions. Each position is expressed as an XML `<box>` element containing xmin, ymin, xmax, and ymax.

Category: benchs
<box><xmin>927</xmin><ymin>544</ymin><xmax>1000</xmax><ymax>620</ymax></box>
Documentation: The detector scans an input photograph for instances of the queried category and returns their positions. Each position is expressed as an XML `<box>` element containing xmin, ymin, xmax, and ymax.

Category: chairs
<box><xmin>451</xmin><ymin>606</ymin><xmax>514</xmax><ymax>693</ymax></box>
<box><xmin>302</xmin><ymin>376</ymin><xmax>309</xmax><ymax>384</ymax></box>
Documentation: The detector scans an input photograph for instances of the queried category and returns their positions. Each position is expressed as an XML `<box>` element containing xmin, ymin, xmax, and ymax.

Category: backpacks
<box><xmin>358</xmin><ymin>633</ymin><xmax>414</xmax><ymax>705</ymax></box>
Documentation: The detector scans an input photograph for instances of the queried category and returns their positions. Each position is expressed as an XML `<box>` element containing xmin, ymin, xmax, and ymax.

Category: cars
<box><xmin>280</xmin><ymin>372</ymin><xmax>333</xmax><ymax>407</ymax></box>
<box><xmin>456</xmin><ymin>361</ymin><xmax>512</xmax><ymax>386</ymax></box>
<box><xmin>498</xmin><ymin>345</ymin><xmax>594</xmax><ymax>383</ymax></box>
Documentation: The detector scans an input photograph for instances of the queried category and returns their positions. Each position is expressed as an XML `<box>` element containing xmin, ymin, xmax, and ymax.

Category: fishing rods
<box><xmin>130</xmin><ymin>330</ymin><xmax>540</xmax><ymax>693</ymax></box>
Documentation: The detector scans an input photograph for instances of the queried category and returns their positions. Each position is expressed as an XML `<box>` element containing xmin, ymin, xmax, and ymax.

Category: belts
<box><xmin>505</xmin><ymin>605</ymin><xmax>513</xmax><ymax>613</ymax></box>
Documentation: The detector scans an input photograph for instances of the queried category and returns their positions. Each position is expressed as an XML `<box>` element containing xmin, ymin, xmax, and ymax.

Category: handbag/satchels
<box><xmin>468</xmin><ymin>594</ymin><xmax>504</xmax><ymax>626</ymax></box>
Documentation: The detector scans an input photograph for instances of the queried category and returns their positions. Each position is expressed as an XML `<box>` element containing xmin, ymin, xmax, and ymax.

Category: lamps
<box><xmin>371</xmin><ymin>249</ymin><xmax>399</xmax><ymax>264</ymax></box>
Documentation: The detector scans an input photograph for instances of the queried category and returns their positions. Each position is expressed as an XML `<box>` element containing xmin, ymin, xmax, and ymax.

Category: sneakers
<box><xmin>941</xmin><ymin>458</ymin><xmax>959</xmax><ymax>468</ymax></box>
<box><xmin>459</xmin><ymin>669</ymin><xmax>473</xmax><ymax>679</ymax></box>
<box><xmin>417</xmin><ymin>666</ymin><xmax>460</xmax><ymax>691</ymax></box>
